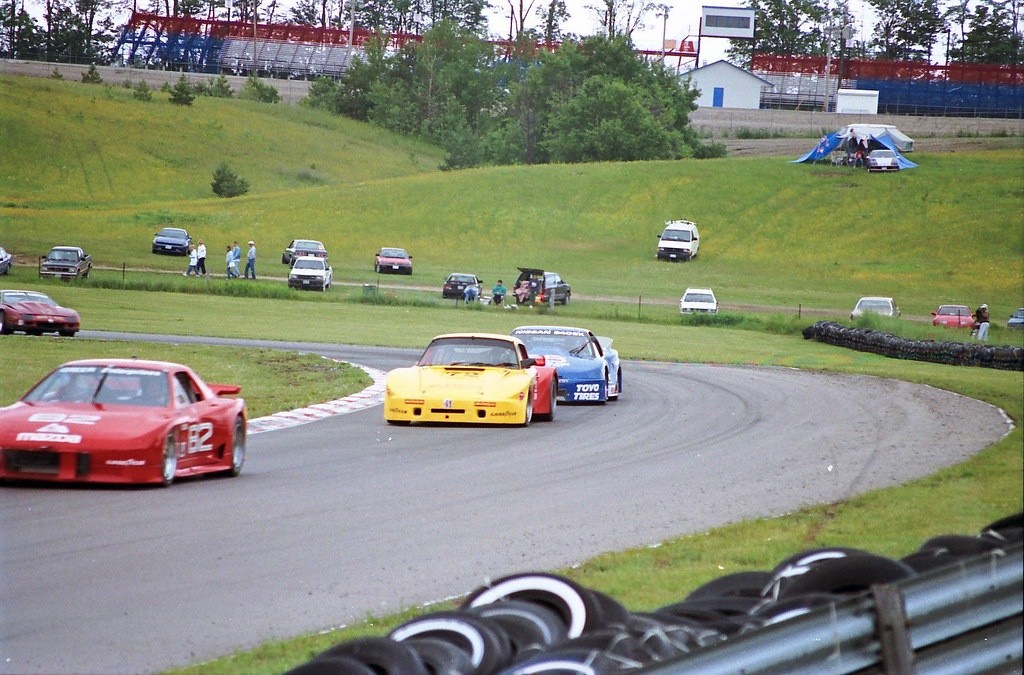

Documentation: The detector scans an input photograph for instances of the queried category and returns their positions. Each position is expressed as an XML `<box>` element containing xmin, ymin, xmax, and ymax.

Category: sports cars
<box><xmin>0</xmin><ymin>289</ymin><xmax>81</xmax><ymax>338</ymax></box>
<box><xmin>0</xmin><ymin>358</ymin><xmax>248</xmax><ymax>489</ymax></box>
<box><xmin>500</xmin><ymin>326</ymin><xmax>622</xmax><ymax>406</ymax></box>
<box><xmin>383</xmin><ymin>332</ymin><xmax>559</xmax><ymax>430</ymax></box>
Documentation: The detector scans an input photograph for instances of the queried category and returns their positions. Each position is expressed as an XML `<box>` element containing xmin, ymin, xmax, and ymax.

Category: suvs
<box><xmin>680</xmin><ymin>287</ymin><xmax>718</xmax><ymax>316</ymax></box>
<box><xmin>513</xmin><ymin>266</ymin><xmax>572</xmax><ymax>306</ymax></box>
<box><xmin>657</xmin><ymin>219</ymin><xmax>700</xmax><ymax>262</ymax></box>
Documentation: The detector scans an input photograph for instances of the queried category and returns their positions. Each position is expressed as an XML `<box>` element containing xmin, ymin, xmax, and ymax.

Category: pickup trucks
<box><xmin>40</xmin><ymin>246</ymin><xmax>93</xmax><ymax>282</ymax></box>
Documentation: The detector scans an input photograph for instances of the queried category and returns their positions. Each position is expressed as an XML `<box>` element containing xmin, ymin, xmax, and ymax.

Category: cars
<box><xmin>1007</xmin><ymin>307</ymin><xmax>1024</xmax><ymax>329</ymax></box>
<box><xmin>282</xmin><ymin>239</ymin><xmax>328</xmax><ymax>269</ymax></box>
<box><xmin>931</xmin><ymin>305</ymin><xmax>977</xmax><ymax>329</ymax></box>
<box><xmin>850</xmin><ymin>296</ymin><xmax>901</xmax><ymax>322</ymax></box>
<box><xmin>442</xmin><ymin>273</ymin><xmax>483</xmax><ymax>301</ymax></box>
<box><xmin>374</xmin><ymin>247</ymin><xmax>413</xmax><ymax>275</ymax></box>
<box><xmin>288</xmin><ymin>256</ymin><xmax>333</xmax><ymax>292</ymax></box>
<box><xmin>0</xmin><ymin>247</ymin><xmax>13</xmax><ymax>275</ymax></box>
<box><xmin>865</xmin><ymin>149</ymin><xmax>901</xmax><ymax>173</ymax></box>
<box><xmin>152</xmin><ymin>228</ymin><xmax>192</xmax><ymax>256</ymax></box>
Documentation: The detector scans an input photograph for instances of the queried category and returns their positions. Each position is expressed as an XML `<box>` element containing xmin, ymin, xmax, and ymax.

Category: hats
<box><xmin>980</xmin><ymin>306</ymin><xmax>985</xmax><ymax>309</ymax></box>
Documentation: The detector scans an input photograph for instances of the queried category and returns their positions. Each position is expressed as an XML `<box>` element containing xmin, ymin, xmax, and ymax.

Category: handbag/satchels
<box><xmin>228</xmin><ymin>262</ymin><xmax>235</xmax><ymax>267</ymax></box>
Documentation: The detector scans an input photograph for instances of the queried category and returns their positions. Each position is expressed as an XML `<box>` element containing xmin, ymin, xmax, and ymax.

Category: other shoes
<box><xmin>196</xmin><ymin>275</ymin><xmax>200</xmax><ymax>277</ymax></box>
<box><xmin>183</xmin><ymin>273</ymin><xmax>188</xmax><ymax>276</ymax></box>
<box><xmin>201</xmin><ymin>273</ymin><xmax>207</xmax><ymax>275</ymax></box>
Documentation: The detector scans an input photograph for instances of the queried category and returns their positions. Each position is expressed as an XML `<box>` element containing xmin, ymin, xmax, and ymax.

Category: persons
<box><xmin>528</xmin><ymin>273</ymin><xmax>540</xmax><ymax>308</ymax></box>
<box><xmin>492</xmin><ymin>280</ymin><xmax>506</xmax><ymax>304</ymax></box>
<box><xmin>972</xmin><ymin>304</ymin><xmax>989</xmax><ymax>342</ymax></box>
<box><xmin>857</xmin><ymin>139</ymin><xmax>866</xmax><ymax>158</ymax></box>
<box><xmin>244</xmin><ymin>241</ymin><xmax>256</xmax><ymax>279</ymax></box>
<box><xmin>197</xmin><ymin>239</ymin><xmax>207</xmax><ymax>276</ymax></box>
<box><xmin>182</xmin><ymin>245</ymin><xmax>200</xmax><ymax>277</ymax></box>
<box><xmin>226</xmin><ymin>246</ymin><xmax>237</xmax><ymax>280</ymax></box>
<box><xmin>233</xmin><ymin>242</ymin><xmax>242</xmax><ymax>278</ymax></box>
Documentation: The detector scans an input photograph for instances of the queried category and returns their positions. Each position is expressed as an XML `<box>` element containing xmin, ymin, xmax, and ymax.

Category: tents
<box><xmin>790</xmin><ymin>123</ymin><xmax>919</xmax><ymax>172</ymax></box>
